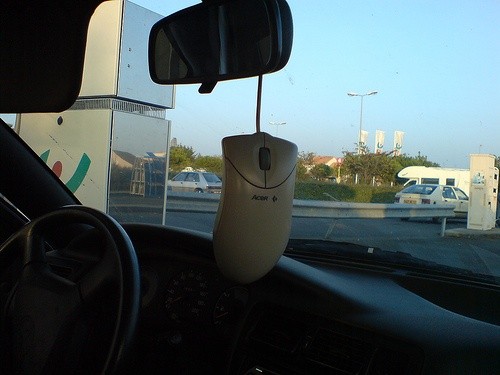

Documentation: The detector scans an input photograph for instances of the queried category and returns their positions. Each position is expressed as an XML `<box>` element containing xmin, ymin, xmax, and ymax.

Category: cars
<box><xmin>167</xmin><ymin>171</ymin><xmax>223</xmax><ymax>195</ymax></box>
<box><xmin>394</xmin><ymin>185</ymin><xmax>469</xmax><ymax>224</ymax></box>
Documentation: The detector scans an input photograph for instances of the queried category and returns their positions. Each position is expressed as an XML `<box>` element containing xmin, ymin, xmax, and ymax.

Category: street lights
<box><xmin>347</xmin><ymin>90</ymin><xmax>378</xmax><ymax>184</ymax></box>
<box><xmin>268</xmin><ymin>122</ymin><xmax>286</xmax><ymax>137</ymax></box>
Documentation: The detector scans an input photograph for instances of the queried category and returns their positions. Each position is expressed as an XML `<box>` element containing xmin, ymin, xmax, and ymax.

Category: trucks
<box><xmin>398</xmin><ymin>166</ymin><xmax>470</xmax><ymax>196</ymax></box>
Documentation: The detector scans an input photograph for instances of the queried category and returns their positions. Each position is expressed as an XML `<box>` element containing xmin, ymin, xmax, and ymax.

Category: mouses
<box><xmin>212</xmin><ymin>133</ymin><xmax>298</xmax><ymax>285</ymax></box>
